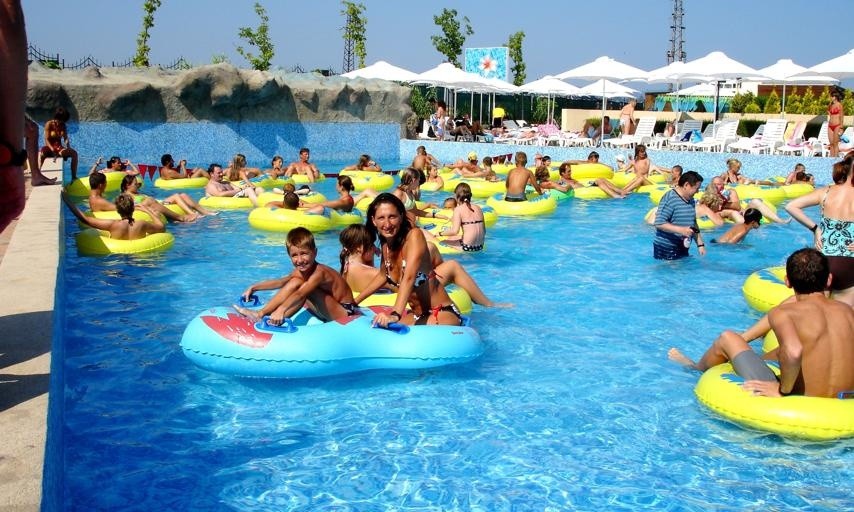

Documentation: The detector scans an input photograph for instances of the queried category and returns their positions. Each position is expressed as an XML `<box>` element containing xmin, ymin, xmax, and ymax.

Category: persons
<box><xmin>0</xmin><ymin>0</ymin><xmax>25</xmax><ymax>234</ymax></box>
<box><xmin>40</xmin><ymin>105</ymin><xmax>79</xmax><ymax>183</ymax></box>
<box><xmin>59</xmin><ymin>145</ymin><xmax>854</xmax><ymax>399</ymax></box>
<box><xmin>24</xmin><ymin>116</ymin><xmax>58</xmax><ymax>186</ymax></box>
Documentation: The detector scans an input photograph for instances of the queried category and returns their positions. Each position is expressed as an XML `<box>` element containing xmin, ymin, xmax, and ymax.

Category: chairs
<box><xmin>603</xmin><ymin>115</ymin><xmax>853</xmax><ymax>159</ymax></box>
<box><xmin>418</xmin><ymin>115</ymin><xmax>596</xmax><ymax>149</ymax></box>
